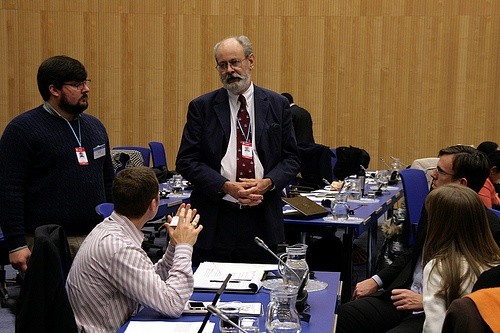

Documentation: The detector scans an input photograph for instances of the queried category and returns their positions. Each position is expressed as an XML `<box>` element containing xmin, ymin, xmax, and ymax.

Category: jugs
<box><xmin>169</xmin><ymin>175</ymin><xmax>183</xmax><ymax>195</ymax></box>
<box><xmin>278</xmin><ymin>243</ymin><xmax>309</xmax><ymax>290</ymax></box>
<box><xmin>266</xmin><ymin>286</ymin><xmax>302</xmax><ymax>333</ymax></box>
<box><xmin>321</xmin><ymin>159</ymin><xmax>402</xmax><ymax>218</ymax></box>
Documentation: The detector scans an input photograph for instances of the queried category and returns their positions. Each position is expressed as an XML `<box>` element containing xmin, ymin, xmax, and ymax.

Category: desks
<box><xmin>282</xmin><ymin>170</ymin><xmax>405</xmax><ymax>277</ymax></box>
<box><xmin>151</xmin><ymin>181</ymin><xmax>192</xmax><ymax>243</ymax></box>
<box><xmin>114</xmin><ymin>270</ymin><xmax>341</xmax><ymax>333</ymax></box>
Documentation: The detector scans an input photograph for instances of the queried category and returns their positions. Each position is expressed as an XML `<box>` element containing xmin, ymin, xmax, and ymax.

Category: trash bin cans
<box><xmin>329</xmin><ymin>243</ymin><xmax>368</xmax><ymax>296</ymax></box>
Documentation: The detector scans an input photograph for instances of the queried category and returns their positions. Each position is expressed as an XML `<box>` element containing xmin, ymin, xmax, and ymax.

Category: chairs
<box><xmin>398</xmin><ymin>168</ymin><xmax>429</xmax><ymax>246</ymax></box>
<box><xmin>109</xmin><ymin>142</ymin><xmax>169</xmax><ymax>173</ymax></box>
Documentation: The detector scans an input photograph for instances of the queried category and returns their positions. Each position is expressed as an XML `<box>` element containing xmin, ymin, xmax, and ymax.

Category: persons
<box><xmin>422</xmin><ymin>184</ymin><xmax>500</xmax><ymax>333</ymax></box>
<box><xmin>173</xmin><ymin>35</ymin><xmax>299</xmax><ymax>264</ymax></box>
<box><xmin>65</xmin><ymin>165</ymin><xmax>203</xmax><ymax>333</ymax></box>
<box><xmin>0</xmin><ymin>55</ymin><xmax>117</xmax><ymax>333</ymax></box>
<box><xmin>280</xmin><ymin>91</ymin><xmax>316</xmax><ymax>190</ymax></box>
<box><xmin>340</xmin><ymin>141</ymin><xmax>500</xmax><ymax>333</ymax></box>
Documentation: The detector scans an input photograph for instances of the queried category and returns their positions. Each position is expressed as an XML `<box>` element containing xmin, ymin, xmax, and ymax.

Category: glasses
<box><xmin>216</xmin><ymin>51</ymin><xmax>252</xmax><ymax>73</ymax></box>
<box><xmin>435</xmin><ymin>166</ymin><xmax>452</xmax><ymax>176</ymax></box>
<box><xmin>64</xmin><ymin>79</ymin><xmax>91</xmax><ymax>93</ymax></box>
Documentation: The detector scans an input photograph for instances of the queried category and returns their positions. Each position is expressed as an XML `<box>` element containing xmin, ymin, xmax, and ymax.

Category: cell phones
<box><xmin>188</xmin><ymin>301</ymin><xmax>206</xmax><ymax>310</ymax></box>
<box><xmin>218</xmin><ymin>316</ymin><xmax>239</xmax><ymax>331</ymax></box>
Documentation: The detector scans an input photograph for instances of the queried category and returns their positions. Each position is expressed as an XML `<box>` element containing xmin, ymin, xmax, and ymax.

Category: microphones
<box><xmin>390</xmin><ymin>155</ymin><xmax>411</xmax><ymax>169</ymax></box>
<box><xmin>207</xmin><ymin>304</ymin><xmax>248</xmax><ymax>333</ymax></box>
<box><xmin>359</xmin><ymin>164</ymin><xmax>384</xmax><ymax>196</ymax></box>
<box><xmin>378</xmin><ymin>157</ymin><xmax>399</xmax><ymax>186</ymax></box>
<box><xmin>254</xmin><ymin>236</ymin><xmax>310</xmax><ymax>312</ymax></box>
<box><xmin>322</xmin><ymin>177</ymin><xmax>351</xmax><ymax>214</ymax></box>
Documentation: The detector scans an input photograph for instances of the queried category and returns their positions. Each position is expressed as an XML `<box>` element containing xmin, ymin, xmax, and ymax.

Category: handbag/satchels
<box><xmin>333</xmin><ymin>145</ymin><xmax>371</xmax><ymax>181</ymax></box>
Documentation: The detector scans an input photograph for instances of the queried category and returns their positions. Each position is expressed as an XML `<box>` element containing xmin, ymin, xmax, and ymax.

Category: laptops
<box><xmin>283</xmin><ymin>196</ymin><xmax>329</xmax><ymax>220</ymax></box>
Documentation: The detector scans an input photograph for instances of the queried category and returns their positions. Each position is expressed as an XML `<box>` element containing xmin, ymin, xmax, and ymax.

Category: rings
<box><xmin>190</xmin><ymin>222</ymin><xmax>197</xmax><ymax>228</ymax></box>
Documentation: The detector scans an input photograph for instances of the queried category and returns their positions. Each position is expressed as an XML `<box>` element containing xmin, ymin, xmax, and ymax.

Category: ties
<box><xmin>236</xmin><ymin>95</ymin><xmax>256</xmax><ymax>182</ymax></box>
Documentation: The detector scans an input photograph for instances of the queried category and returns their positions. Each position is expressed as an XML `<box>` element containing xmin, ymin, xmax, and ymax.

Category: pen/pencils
<box><xmin>210</xmin><ymin>280</ymin><xmax>239</xmax><ymax>283</ymax></box>
<box><xmin>204</xmin><ymin>308</ymin><xmax>235</xmax><ymax>310</ymax></box>
